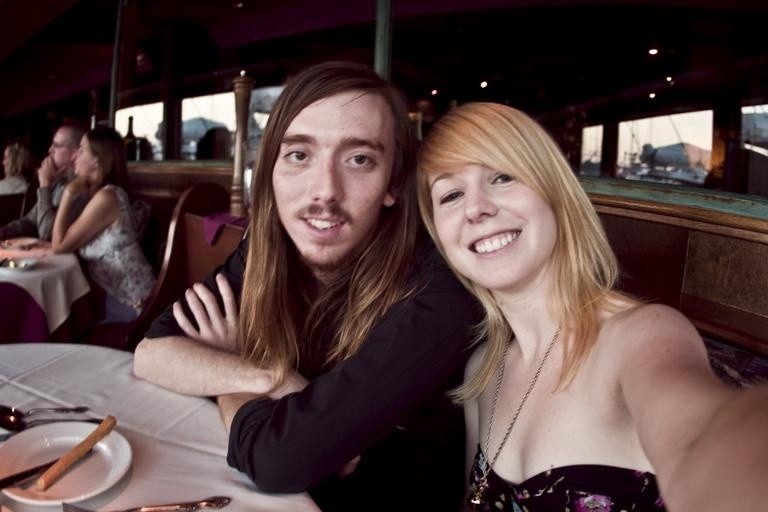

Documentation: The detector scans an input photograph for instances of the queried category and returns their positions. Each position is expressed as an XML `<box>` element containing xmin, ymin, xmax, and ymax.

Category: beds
<box><xmin>181</xmin><ymin>117</ymin><xmax>226</xmax><ymax>142</ymax></box>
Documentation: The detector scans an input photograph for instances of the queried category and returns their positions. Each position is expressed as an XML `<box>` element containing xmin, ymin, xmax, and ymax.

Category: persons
<box><xmin>49</xmin><ymin>126</ymin><xmax>156</xmax><ymax>346</ymax></box>
<box><xmin>1</xmin><ymin>143</ymin><xmax>32</xmax><ymax>197</ymax></box>
<box><xmin>195</xmin><ymin>126</ymin><xmax>233</xmax><ymax>161</ymax></box>
<box><xmin>416</xmin><ymin>101</ymin><xmax>768</xmax><ymax>512</ymax></box>
<box><xmin>133</xmin><ymin>64</ymin><xmax>485</xmax><ymax>512</ymax></box>
<box><xmin>1</xmin><ymin>123</ymin><xmax>94</xmax><ymax>245</ymax></box>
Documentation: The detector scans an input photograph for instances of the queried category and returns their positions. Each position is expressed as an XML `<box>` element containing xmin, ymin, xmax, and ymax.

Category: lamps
<box><xmin>117</xmin><ymin>181</ymin><xmax>232</xmax><ymax>347</ymax></box>
<box><xmin>0</xmin><ymin>181</ymin><xmax>39</xmax><ymax>229</ymax></box>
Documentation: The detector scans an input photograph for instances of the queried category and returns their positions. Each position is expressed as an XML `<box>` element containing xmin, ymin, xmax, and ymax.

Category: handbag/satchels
<box><xmin>66</xmin><ymin>187</ymin><xmax>75</xmax><ymax>196</ymax></box>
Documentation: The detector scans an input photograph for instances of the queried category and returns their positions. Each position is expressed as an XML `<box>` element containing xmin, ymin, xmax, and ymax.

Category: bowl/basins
<box><xmin>470</xmin><ymin>316</ymin><xmax>567</xmax><ymax>504</ymax></box>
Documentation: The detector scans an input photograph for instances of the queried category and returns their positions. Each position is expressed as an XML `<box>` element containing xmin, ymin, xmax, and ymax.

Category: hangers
<box><xmin>0</xmin><ymin>404</ymin><xmax>87</xmax><ymax>424</ymax></box>
<box><xmin>1</xmin><ymin>417</ymin><xmax>103</xmax><ymax>433</ymax></box>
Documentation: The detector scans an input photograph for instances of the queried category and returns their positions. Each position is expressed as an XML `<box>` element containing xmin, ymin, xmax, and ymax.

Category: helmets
<box><xmin>0</xmin><ymin>257</ymin><xmax>38</xmax><ymax>272</ymax></box>
<box><xmin>1</xmin><ymin>421</ymin><xmax>135</xmax><ymax>506</ymax></box>
<box><xmin>1</xmin><ymin>237</ymin><xmax>37</xmax><ymax>248</ymax></box>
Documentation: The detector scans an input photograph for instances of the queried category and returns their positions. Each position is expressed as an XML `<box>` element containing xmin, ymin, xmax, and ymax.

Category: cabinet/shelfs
<box><xmin>123</xmin><ymin>116</ymin><xmax>136</xmax><ymax>161</ymax></box>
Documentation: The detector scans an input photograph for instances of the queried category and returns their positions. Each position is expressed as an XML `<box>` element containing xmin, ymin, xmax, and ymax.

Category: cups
<box><xmin>51</xmin><ymin>140</ymin><xmax>79</xmax><ymax>151</ymax></box>
